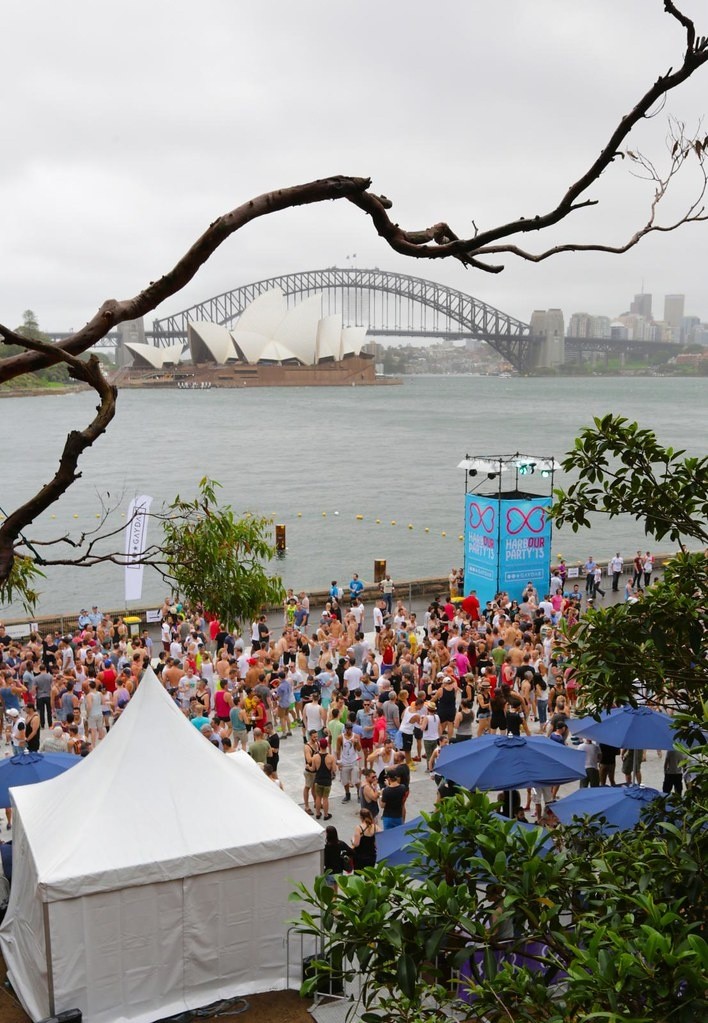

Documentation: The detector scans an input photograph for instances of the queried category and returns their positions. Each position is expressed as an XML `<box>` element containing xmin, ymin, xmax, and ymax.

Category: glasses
<box><xmin>363</xmin><ymin>703</ymin><xmax>371</xmax><ymax>706</ymax></box>
<box><xmin>369</xmin><ymin>774</ymin><xmax>377</xmax><ymax>778</ymax></box>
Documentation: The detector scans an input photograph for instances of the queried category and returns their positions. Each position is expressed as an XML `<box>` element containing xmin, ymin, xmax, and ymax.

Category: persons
<box><xmin>320</xmin><ymin>824</ymin><xmax>353</xmax><ymax>919</ymax></box>
<box><xmin>431</xmin><ymin>543</ymin><xmax>707</xmax><ymax>945</ymax></box>
<box><xmin>352</xmin><ymin>806</ymin><xmax>382</xmax><ymax>868</ymax></box>
<box><xmin>0</xmin><ymin>549</ymin><xmax>432</xmax><ymax>821</ymax></box>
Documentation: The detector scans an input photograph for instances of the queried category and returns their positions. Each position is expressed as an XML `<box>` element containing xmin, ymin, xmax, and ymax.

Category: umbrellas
<box><xmin>433</xmin><ymin>730</ymin><xmax>585</xmax><ymax>823</ymax></box>
<box><xmin>0</xmin><ymin>751</ymin><xmax>84</xmax><ymax>808</ymax></box>
<box><xmin>567</xmin><ymin>705</ymin><xmax>707</xmax><ymax>788</ymax></box>
<box><xmin>374</xmin><ymin>804</ymin><xmax>559</xmax><ymax>918</ymax></box>
<box><xmin>549</xmin><ymin>785</ymin><xmax>692</xmax><ymax>854</ymax></box>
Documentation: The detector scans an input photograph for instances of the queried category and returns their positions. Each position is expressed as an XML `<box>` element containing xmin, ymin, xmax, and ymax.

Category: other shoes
<box><xmin>531</xmin><ymin>811</ymin><xmax>537</xmax><ymax>817</ymax></box>
<box><xmin>523</xmin><ymin>806</ymin><xmax>529</xmax><ymax>810</ymax></box>
<box><xmin>280</xmin><ymin>735</ymin><xmax>287</xmax><ymax>739</ymax></box>
<box><xmin>315</xmin><ymin>811</ymin><xmax>322</xmax><ymax>819</ymax></box>
<box><xmin>278</xmin><ymin>727</ymin><xmax>282</xmax><ymax>731</ymax></box>
<box><xmin>287</xmin><ymin>732</ymin><xmax>292</xmax><ymax>736</ymax></box>
<box><xmin>291</xmin><ymin>722</ymin><xmax>297</xmax><ymax>728</ymax></box>
<box><xmin>314</xmin><ymin>802</ymin><xmax>323</xmax><ymax>808</ymax></box>
<box><xmin>305</xmin><ymin>807</ymin><xmax>314</xmax><ymax>815</ymax></box>
<box><xmin>323</xmin><ymin>813</ymin><xmax>332</xmax><ymax>820</ymax></box>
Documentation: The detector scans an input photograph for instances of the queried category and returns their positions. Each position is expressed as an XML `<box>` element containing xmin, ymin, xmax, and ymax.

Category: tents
<box><xmin>0</xmin><ymin>666</ymin><xmax>325</xmax><ymax>1023</ymax></box>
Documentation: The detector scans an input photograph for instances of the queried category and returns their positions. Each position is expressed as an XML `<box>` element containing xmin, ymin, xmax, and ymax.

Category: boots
<box><xmin>341</xmin><ymin>793</ymin><xmax>351</xmax><ymax>803</ymax></box>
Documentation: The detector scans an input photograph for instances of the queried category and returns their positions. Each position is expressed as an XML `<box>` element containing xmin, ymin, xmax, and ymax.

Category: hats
<box><xmin>319</xmin><ymin>738</ymin><xmax>329</xmax><ymax>747</ymax></box>
<box><xmin>252</xmin><ymin>654</ymin><xmax>260</xmax><ymax>659</ymax></box>
<box><xmin>246</xmin><ymin>658</ymin><xmax>256</xmax><ymax>665</ymax></box>
<box><xmin>444</xmin><ymin>667</ymin><xmax>454</xmax><ymax>676</ymax></box>
<box><xmin>6</xmin><ymin>708</ymin><xmax>18</xmax><ymax>716</ymax></box>
<box><xmin>118</xmin><ymin>698</ymin><xmax>129</xmax><ymax>707</ymax></box>
<box><xmin>563</xmin><ymin>592</ymin><xmax>569</xmax><ymax>597</ymax></box>
<box><xmin>463</xmin><ymin>673</ymin><xmax>474</xmax><ymax>680</ymax></box>
<box><xmin>437</xmin><ymin>672</ymin><xmax>445</xmax><ymax>679</ymax></box>
<box><xmin>443</xmin><ymin>677</ymin><xmax>453</xmax><ymax>684</ymax></box>
<box><xmin>482</xmin><ymin>682</ymin><xmax>492</xmax><ymax>688</ymax></box>
<box><xmin>80</xmin><ymin>608</ymin><xmax>88</xmax><ymax>613</ymax></box>
<box><xmin>426</xmin><ymin>701</ymin><xmax>437</xmax><ymax>709</ymax></box>
<box><xmin>344</xmin><ymin>721</ymin><xmax>354</xmax><ymax>729</ymax></box>
<box><xmin>104</xmin><ymin>659</ymin><xmax>114</xmax><ymax>666</ymax></box>
